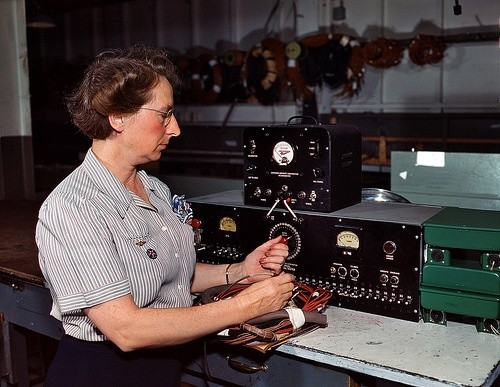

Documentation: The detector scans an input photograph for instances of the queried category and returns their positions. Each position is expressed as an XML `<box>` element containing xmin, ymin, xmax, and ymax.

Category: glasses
<box><xmin>127</xmin><ymin>104</ymin><xmax>174</xmax><ymax>128</ymax></box>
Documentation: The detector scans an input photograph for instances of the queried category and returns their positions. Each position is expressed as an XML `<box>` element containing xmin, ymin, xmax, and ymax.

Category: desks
<box><xmin>0</xmin><ymin>210</ymin><xmax>500</xmax><ymax>387</ymax></box>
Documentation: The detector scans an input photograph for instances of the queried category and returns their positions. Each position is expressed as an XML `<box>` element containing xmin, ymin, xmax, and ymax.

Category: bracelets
<box><xmin>226</xmin><ymin>263</ymin><xmax>232</xmax><ymax>284</ymax></box>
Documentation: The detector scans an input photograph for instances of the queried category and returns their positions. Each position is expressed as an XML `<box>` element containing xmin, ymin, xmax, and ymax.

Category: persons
<box><xmin>34</xmin><ymin>40</ymin><xmax>297</xmax><ymax>386</ymax></box>
